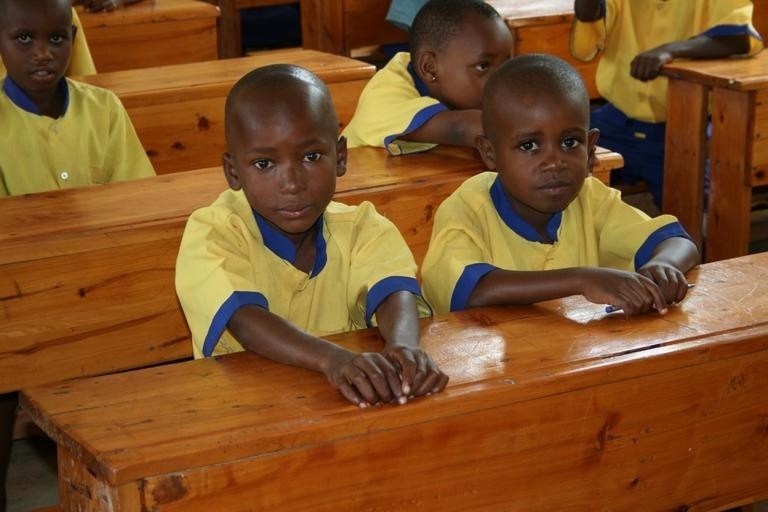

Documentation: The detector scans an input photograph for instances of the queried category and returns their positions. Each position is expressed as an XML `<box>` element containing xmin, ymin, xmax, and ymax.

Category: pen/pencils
<box><xmin>606</xmin><ymin>284</ymin><xmax>695</xmax><ymax>313</ymax></box>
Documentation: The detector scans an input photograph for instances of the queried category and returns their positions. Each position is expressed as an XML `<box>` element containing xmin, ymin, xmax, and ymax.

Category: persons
<box><xmin>0</xmin><ymin>0</ymin><xmax>160</xmax><ymax>200</ymax></box>
<box><xmin>566</xmin><ymin>0</ymin><xmax>767</xmax><ymax>264</ymax></box>
<box><xmin>336</xmin><ymin>0</ymin><xmax>515</xmax><ymax>161</ymax></box>
<box><xmin>416</xmin><ymin>50</ymin><xmax>700</xmax><ymax>317</ymax></box>
<box><xmin>173</xmin><ymin>63</ymin><xmax>451</xmax><ymax>413</ymax></box>
<box><xmin>0</xmin><ymin>0</ymin><xmax>98</xmax><ymax>84</ymax></box>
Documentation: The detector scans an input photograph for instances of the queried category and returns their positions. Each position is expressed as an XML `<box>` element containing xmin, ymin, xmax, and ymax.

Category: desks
<box><xmin>16</xmin><ymin>250</ymin><xmax>768</xmax><ymax>508</ymax></box>
<box><xmin>661</xmin><ymin>50</ymin><xmax>766</xmax><ymax>268</ymax></box>
<box><xmin>2</xmin><ymin>136</ymin><xmax>620</xmax><ymax>392</ymax></box>
<box><xmin>70</xmin><ymin>3</ymin><xmax>614</xmax><ymax>176</ymax></box>
